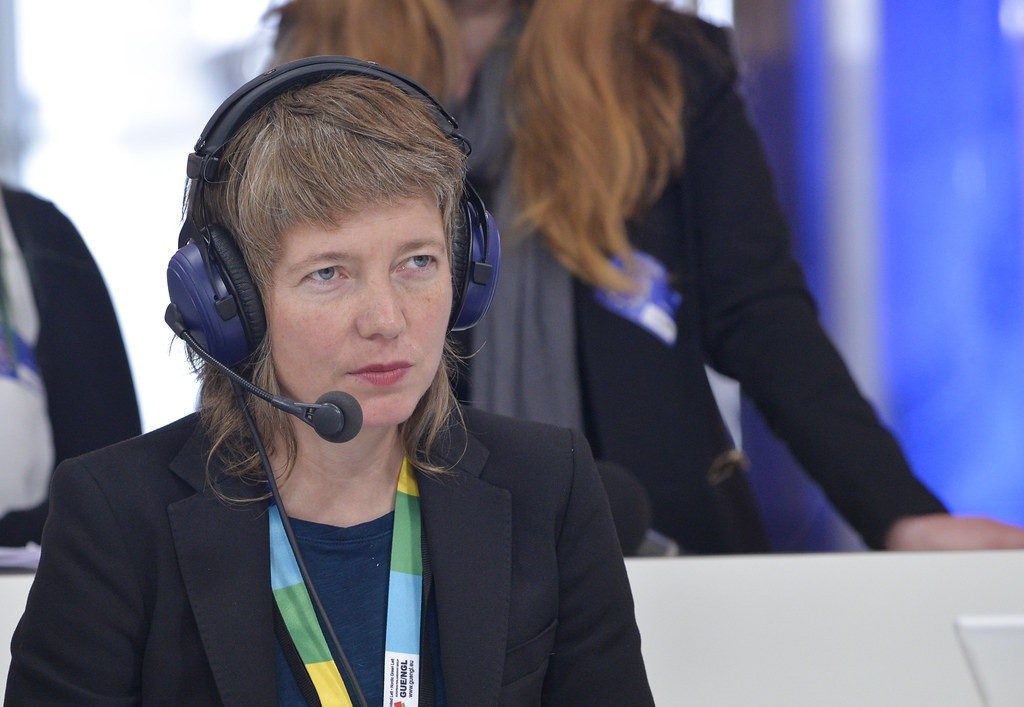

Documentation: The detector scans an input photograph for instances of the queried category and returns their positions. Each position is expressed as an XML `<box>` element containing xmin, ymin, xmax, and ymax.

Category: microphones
<box><xmin>182</xmin><ymin>332</ymin><xmax>365</xmax><ymax>446</ymax></box>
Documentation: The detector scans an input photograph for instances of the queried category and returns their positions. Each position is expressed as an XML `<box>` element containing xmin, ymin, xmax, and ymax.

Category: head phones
<box><xmin>167</xmin><ymin>54</ymin><xmax>501</xmax><ymax>367</ymax></box>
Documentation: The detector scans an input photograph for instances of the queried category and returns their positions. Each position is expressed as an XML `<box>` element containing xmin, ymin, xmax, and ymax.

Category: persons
<box><xmin>3</xmin><ymin>76</ymin><xmax>654</xmax><ymax>707</ymax></box>
<box><xmin>266</xmin><ymin>0</ymin><xmax>1024</xmax><ymax>557</ymax></box>
<box><xmin>1</xmin><ymin>183</ymin><xmax>144</xmax><ymax>568</ymax></box>
